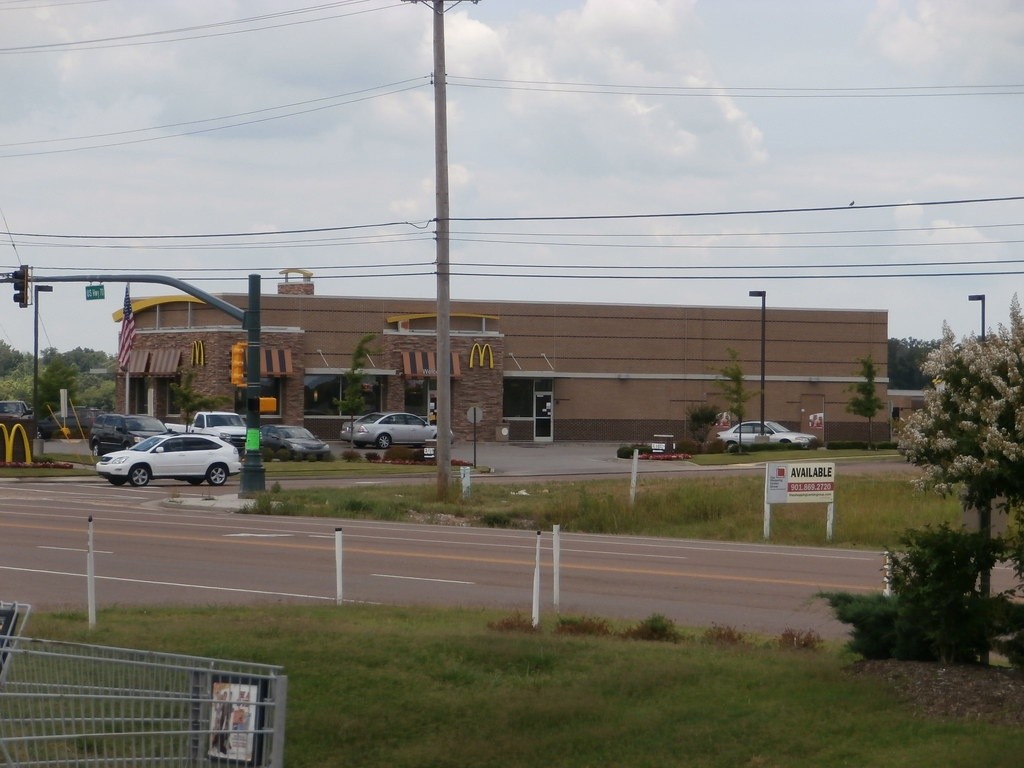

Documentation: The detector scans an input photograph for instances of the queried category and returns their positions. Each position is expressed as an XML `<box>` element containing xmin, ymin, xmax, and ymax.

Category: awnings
<box><xmin>401</xmin><ymin>351</ymin><xmax>462</xmax><ymax>379</ymax></box>
<box><xmin>128</xmin><ymin>350</ymin><xmax>150</xmax><ymax>376</ymax></box>
<box><xmin>150</xmin><ymin>349</ymin><xmax>180</xmax><ymax>376</ymax></box>
<box><xmin>259</xmin><ymin>349</ymin><xmax>292</xmax><ymax>377</ymax></box>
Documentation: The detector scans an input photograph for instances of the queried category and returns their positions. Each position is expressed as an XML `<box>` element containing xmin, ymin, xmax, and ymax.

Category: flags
<box><xmin>118</xmin><ymin>287</ymin><xmax>136</xmax><ymax>373</ymax></box>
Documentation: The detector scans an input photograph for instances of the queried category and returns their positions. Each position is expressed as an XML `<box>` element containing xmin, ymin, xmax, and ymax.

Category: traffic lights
<box><xmin>10</xmin><ymin>269</ymin><xmax>29</xmax><ymax>308</ymax></box>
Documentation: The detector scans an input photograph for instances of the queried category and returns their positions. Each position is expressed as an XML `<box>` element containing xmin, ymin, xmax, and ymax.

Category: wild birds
<box><xmin>849</xmin><ymin>201</ymin><xmax>855</xmax><ymax>206</ymax></box>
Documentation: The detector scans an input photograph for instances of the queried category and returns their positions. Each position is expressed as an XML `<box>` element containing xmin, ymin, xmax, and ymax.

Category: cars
<box><xmin>340</xmin><ymin>410</ymin><xmax>455</xmax><ymax>450</ymax></box>
<box><xmin>715</xmin><ymin>420</ymin><xmax>818</xmax><ymax>450</ymax></box>
<box><xmin>37</xmin><ymin>409</ymin><xmax>96</xmax><ymax>439</ymax></box>
<box><xmin>259</xmin><ymin>424</ymin><xmax>331</xmax><ymax>463</ymax></box>
<box><xmin>0</xmin><ymin>400</ymin><xmax>33</xmax><ymax>424</ymax></box>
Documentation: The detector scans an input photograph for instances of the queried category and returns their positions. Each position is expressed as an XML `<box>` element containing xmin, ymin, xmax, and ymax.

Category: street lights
<box><xmin>967</xmin><ymin>293</ymin><xmax>988</xmax><ymax>343</ymax></box>
<box><xmin>749</xmin><ymin>290</ymin><xmax>771</xmax><ymax>446</ymax></box>
<box><xmin>33</xmin><ymin>283</ymin><xmax>54</xmax><ymax>439</ymax></box>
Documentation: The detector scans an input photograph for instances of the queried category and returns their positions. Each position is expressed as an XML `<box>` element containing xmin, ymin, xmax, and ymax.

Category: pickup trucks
<box><xmin>165</xmin><ymin>411</ymin><xmax>247</xmax><ymax>454</ymax></box>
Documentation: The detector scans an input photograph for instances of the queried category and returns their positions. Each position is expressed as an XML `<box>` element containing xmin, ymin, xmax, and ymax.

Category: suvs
<box><xmin>96</xmin><ymin>431</ymin><xmax>242</xmax><ymax>487</ymax></box>
<box><xmin>86</xmin><ymin>413</ymin><xmax>178</xmax><ymax>457</ymax></box>
